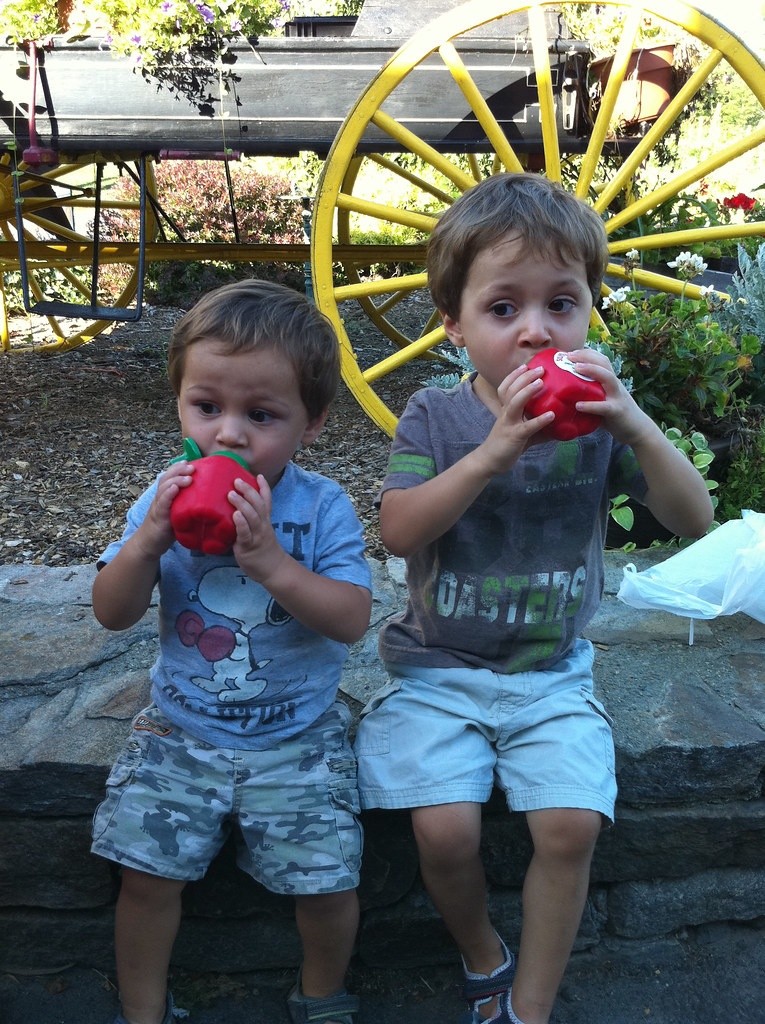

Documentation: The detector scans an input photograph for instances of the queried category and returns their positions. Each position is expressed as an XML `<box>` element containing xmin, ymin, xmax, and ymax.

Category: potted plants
<box><xmin>586</xmin><ymin>4</ymin><xmax>676</xmax><ymax>130</ymax></box>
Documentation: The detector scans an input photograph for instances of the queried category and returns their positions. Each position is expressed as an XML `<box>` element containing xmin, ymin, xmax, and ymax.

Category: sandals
<box><xmin>112</xmin><ymin>989</ymin><xmax>173</xmax><ymax>1023</ymax></box>
<box><xmin>456</xmin><ymin>928</ymin><xmax>521</xmax><ymax>1024</ymax></box>
<box><xmin>482</xmin><ymin>987</ymin><xmax>547</xmax><ymax>1024</ymax></box>
<box><xmin>283</xmin><ymin>964</ymin><xmax>360</xmax><ymax>1024</ymax></box>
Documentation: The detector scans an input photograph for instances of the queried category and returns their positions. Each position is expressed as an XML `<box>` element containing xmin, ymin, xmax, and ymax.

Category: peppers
<box><xmin>522</xmin><ymin>347</ymin><xmax>606</xmax><ymax>442</ymax></box>
<box><xmin>164</xmin><ymin>439</ymin><xmax>258</xmax><ymax>554</ymax></box>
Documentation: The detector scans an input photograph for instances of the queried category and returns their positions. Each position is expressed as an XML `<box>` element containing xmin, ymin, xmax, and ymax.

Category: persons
<box><xmin>90</xmin><ymin>279</ymin><xmax>375</xmax><ymax>1024</ymax></box>
<box><xmin>354</xmin><ymin>174</ymin><xmax>713</xmax><ymax>1024</ymax></box>
